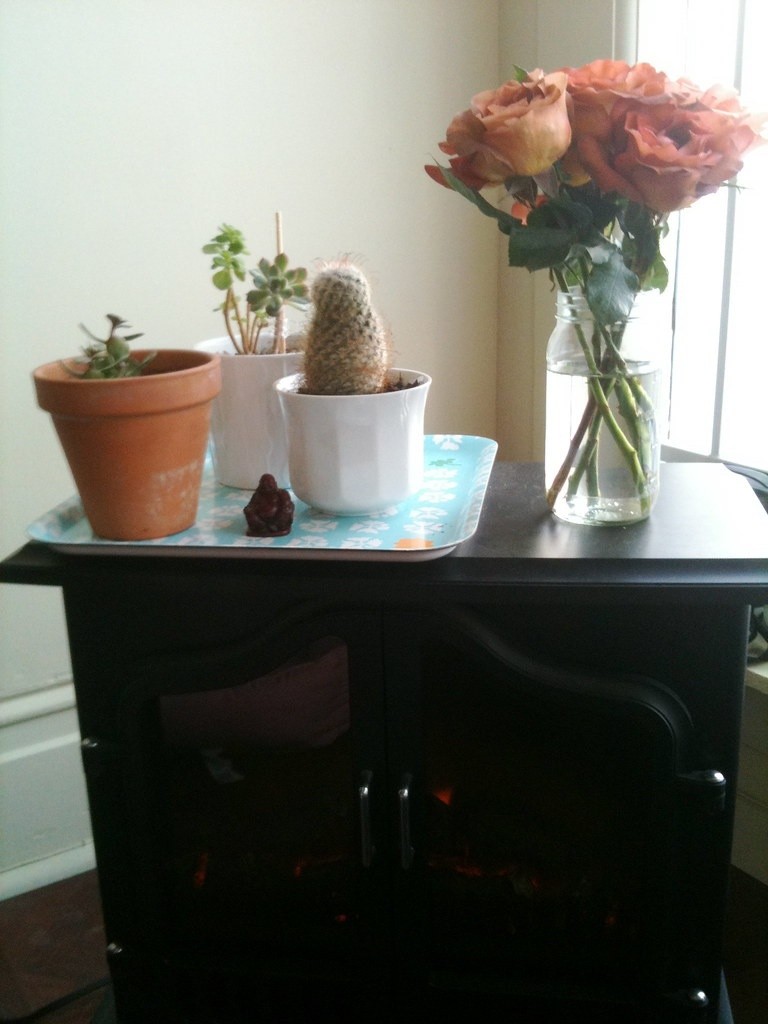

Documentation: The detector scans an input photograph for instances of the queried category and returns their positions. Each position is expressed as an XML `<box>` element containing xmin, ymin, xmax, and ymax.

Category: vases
<box><xmin>544</xmin><ymin>283</ymin><xmax>667</xmax><ymax>528</ymax></box>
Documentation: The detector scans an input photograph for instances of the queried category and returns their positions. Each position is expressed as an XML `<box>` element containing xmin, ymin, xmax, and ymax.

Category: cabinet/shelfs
<box><xmin>0</xmin><ymin>460</ymin><xmax>768</xmax><ymax>1024</ymax></box>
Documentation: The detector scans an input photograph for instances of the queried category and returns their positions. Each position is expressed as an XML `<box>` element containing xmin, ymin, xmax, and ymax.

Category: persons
<box><xmin>244</xmin><ymin>474</ymin><xmax>295</xmax><ymax>533</ymax></box>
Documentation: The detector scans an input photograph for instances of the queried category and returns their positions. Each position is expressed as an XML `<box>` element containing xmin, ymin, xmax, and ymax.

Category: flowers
<box><xmin>420</xmin><ymin>55</ymin><xmax>768</xmax><ymax>328</ymax></box>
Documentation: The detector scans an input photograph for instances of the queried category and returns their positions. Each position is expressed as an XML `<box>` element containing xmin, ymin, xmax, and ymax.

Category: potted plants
<box><xmin>29</xmin><ymin>311</ymin><xmax>225</xmax><ymax>541</ymax></box>
<box><xmin>272</xmin><ymin>252</ymin><xmax>433</xmax><ymax>520</ymax></box>
<box><xmin>190</xmin><ymin>210</ymin><xmax>308</xmax><ymax>490</ymax></box>
